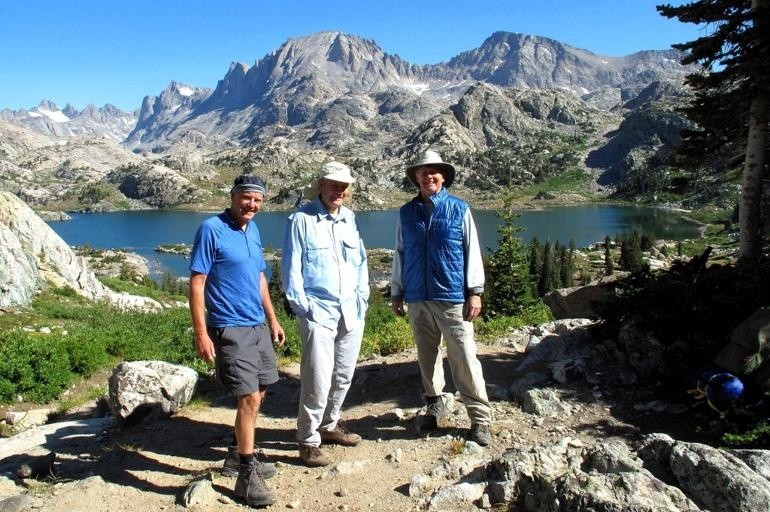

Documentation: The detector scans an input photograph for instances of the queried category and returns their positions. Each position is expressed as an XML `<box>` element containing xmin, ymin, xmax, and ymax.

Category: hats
<box><xmin>229</xmin><ymin>172</ymin><xmax>267</xmax><ymax>198</ymax></box>
<box><xmin>318</xmin><ymin>160</ymin><xmax>357</xmax><ymax>184</ymax></box>
<box><xmin>403</xmin><ymin>149</ymin><xmax>454</xmax><ymax>188</ymax></box>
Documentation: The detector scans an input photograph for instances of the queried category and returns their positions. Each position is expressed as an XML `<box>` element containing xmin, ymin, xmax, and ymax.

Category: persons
<box><xmin>386</xmin><ymin>149</ymin><xmax>494</xmax><ymax>447</ymax></box>
<box><xmin>280</xmin><ymin>160</ymin><xmax>371</xmax><ymax>468</ymax></box>
<box><xmin>188</xmin><ymin>174</ymin><xmax>289</xmax><ymax>507</ymax></box>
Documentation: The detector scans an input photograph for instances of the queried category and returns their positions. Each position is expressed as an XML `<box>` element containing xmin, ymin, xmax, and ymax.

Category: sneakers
<box><xmin>234</xmin><ymin>464</ymin><xmax>274</xmax><ymax>507</ymax></box>
<box><xmin>220</xmin><ymin>445</ymin><xmax>277</xmax><ymax>479</ymax></box>
<box><xmin>415</xmin><ymin>392</ymin><xmax>451</xmax><ymax>435</ymax></box>
<box><xmin>297</xmin><ymin>441</ymin><xmax>334</xmax><ymax>468</ymax></box>
<box><xmin>318</xmin><ymin>419</ymin><xmax>363</xmax><ymax>446</ymax></box>
<box><xmin>468</xmin><ymin>421</ymin><xmax>492</xmax><ymax>445</ymax></box>
